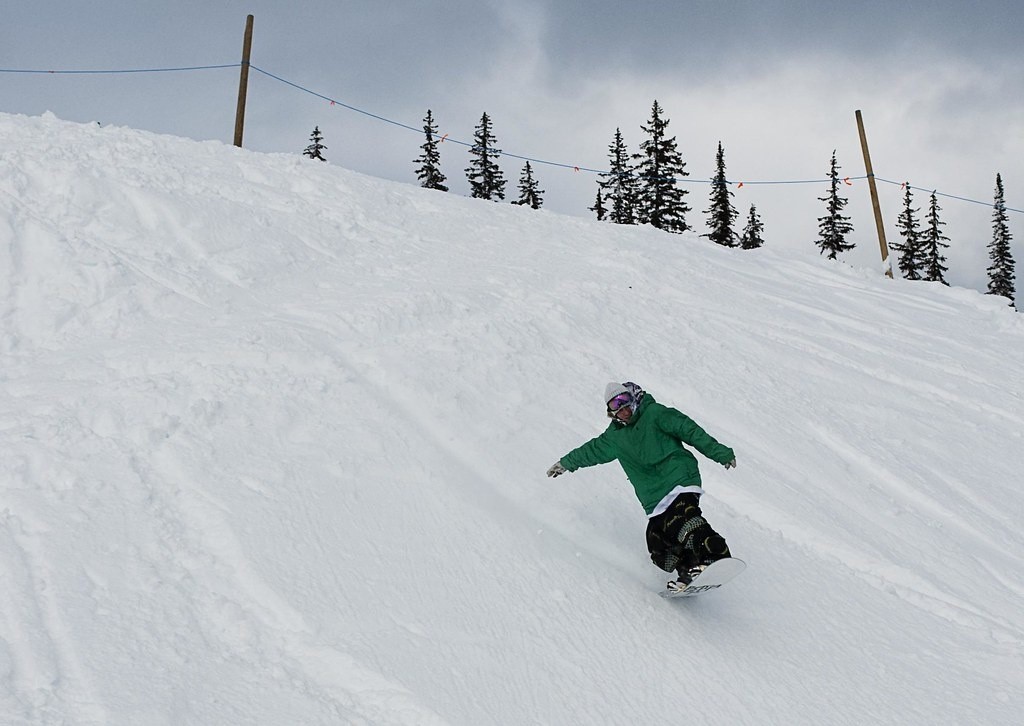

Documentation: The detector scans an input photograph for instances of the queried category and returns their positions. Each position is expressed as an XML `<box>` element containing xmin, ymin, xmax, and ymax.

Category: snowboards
<box><xmin>657</xmin><ymin>557</ymin><xmax>748</xmax><ymax>598</ymax></box>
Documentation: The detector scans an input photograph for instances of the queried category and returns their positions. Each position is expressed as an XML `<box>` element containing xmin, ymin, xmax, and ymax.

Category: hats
<box><xmin>604</xmin><ymin>382</ymin><xmax>634</xmax><ymax>416</ymax></box>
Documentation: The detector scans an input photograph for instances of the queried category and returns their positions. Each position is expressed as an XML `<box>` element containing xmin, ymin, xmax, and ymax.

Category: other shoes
<box><xmin>690</xmin><ymin>568</ymin><xmax>703</xmax><ymax>580</ymax></box>
<box><xmin>667</xmin><ymin>581</ymin><xmax>686</xmax><ymax>594</ymax></box>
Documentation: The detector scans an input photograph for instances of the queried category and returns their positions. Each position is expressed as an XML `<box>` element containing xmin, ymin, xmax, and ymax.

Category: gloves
<box><xmin>546</xmin><ymin>461</ymin><xmax>567</xmax><ymax>478</ymax></box>
<box><xmin>725</xmin><ymin>459</ymin><xmax>736</xmax><ymax>469</ymax></box>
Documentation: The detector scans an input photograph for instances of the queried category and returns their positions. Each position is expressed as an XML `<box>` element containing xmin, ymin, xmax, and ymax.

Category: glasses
<box><xmin>606</xmin><ymin>391</ymin><xmax>633</xmax><ymax>412</ymax></box>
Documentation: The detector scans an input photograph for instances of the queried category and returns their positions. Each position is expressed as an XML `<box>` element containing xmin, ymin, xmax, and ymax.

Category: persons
<box><xmin>546</xmin><ymin>381</ymin><xmax>736</xmax><ymax>591</ymax></box>
